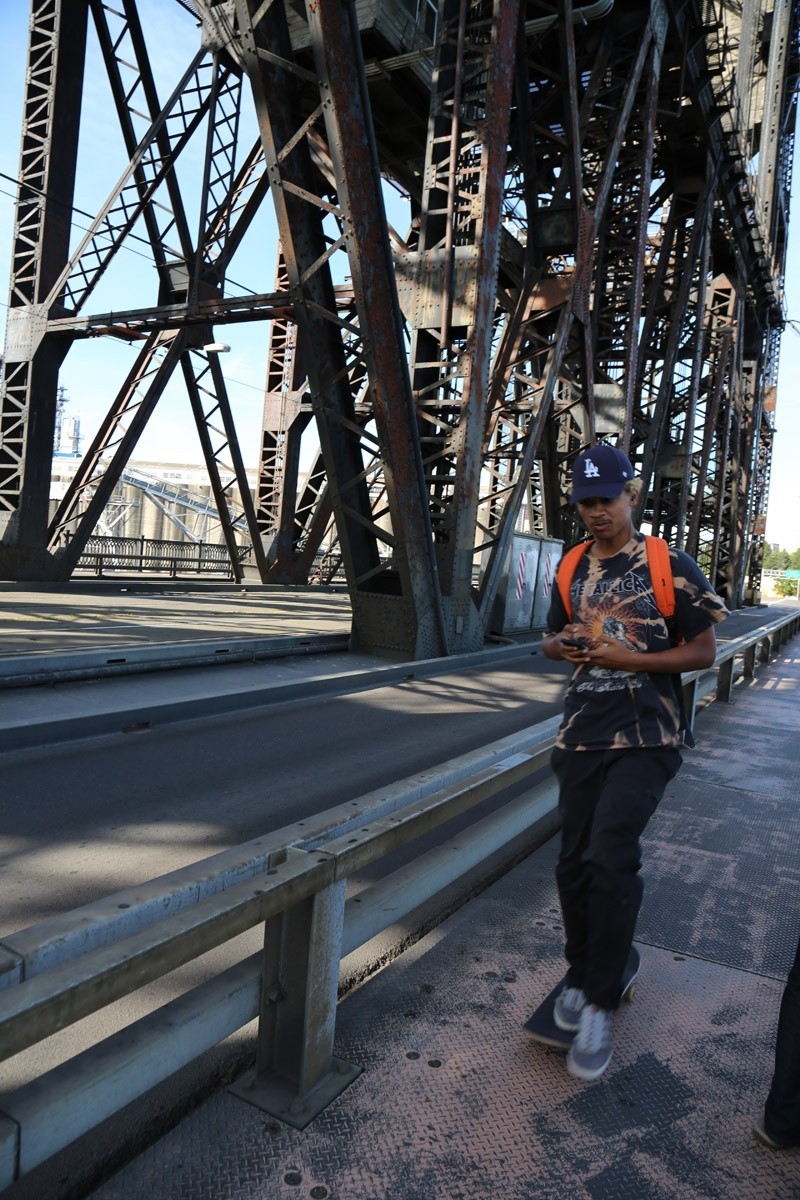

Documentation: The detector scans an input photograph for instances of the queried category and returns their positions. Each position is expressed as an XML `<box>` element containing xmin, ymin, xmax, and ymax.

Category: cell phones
<box><xmin>560</xmin><ymin>639</ymin><xmax>586</xmax><ymax>649</ymax></box>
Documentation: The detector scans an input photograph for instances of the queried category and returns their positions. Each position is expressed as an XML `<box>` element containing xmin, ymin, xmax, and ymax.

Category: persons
<box><xmin>753</xmin><ymin>930</ymin><xmax>800</xmax><ymax>1151</ymax></box>
<box><xmin>543</xmin><ymin>445</ymin><xmax>730</xmax><ymax>1080</ymax></box>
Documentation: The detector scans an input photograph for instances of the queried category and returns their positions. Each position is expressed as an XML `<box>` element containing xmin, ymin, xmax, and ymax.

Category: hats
<box><xmin>570</xmin><ymin>445</ymin><xmax>634</xmax><ymax>503</ymax></box>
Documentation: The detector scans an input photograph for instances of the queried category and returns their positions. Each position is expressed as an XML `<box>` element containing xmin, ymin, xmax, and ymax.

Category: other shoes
<box><xmin>755</xmin><ymin>1114</ymin><xmax>785</xmax><ymax>1150</ymax></box>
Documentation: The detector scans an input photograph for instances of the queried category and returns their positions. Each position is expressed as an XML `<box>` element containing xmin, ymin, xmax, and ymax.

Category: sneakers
<box><xmin>566</xmin><ymin>1004</ymin><xmax>616</xmax><ymax>1081</ymax></box>
<box><xmin>555</xmin><ymin>987</ymin><xmax>589</xmax><ymax>1029</ymax></box>
